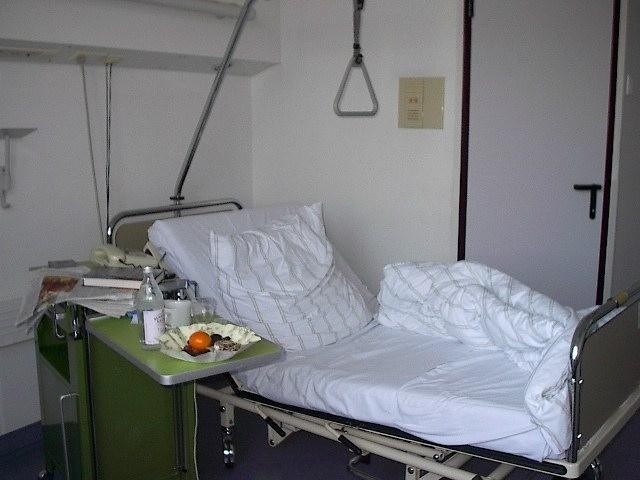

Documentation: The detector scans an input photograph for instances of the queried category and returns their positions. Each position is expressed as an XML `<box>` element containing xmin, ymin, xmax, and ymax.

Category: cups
<box><xmin>163</xmin><ymin>299</ymin><xmax>191</xmax><ymax>327</ymax></box>
<box><xmin>191</xmin><ymin>295</ymin><xmax>217</xmax><ymax>325</ymax></box>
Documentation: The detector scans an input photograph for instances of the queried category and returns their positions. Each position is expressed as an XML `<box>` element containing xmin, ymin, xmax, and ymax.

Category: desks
<box><xmin>33</xmin><ymin>300</ymin><xmax>287</xmax><ymax>480</ymax></box>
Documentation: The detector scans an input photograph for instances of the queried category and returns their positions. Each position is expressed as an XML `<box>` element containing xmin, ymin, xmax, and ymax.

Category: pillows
<box><xmin>209</xmin><ymin>202</ymin><xmax>377</xmax><ymax>352</ymax></box>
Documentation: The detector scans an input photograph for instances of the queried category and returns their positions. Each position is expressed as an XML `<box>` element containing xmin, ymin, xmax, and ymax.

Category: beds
<box><xmin>105</xmin><ymin>198</ymin><xmax>638</xmax><ymax>479</ymax></box>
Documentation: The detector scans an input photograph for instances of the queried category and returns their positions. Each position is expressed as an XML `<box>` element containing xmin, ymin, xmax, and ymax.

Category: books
<box><xmin>81</xmin><ymin>266</ymin><xmax>164</xmax><ymax>290</ymax></box>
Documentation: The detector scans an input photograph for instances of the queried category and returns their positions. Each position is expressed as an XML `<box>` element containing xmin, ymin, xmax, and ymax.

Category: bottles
<box><xmin>135</xmin><ymin>266</ymin><xmax>165</xmax><ymax>346</ymax></box>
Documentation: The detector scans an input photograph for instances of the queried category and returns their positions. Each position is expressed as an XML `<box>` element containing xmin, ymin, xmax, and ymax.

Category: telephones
<box><xmin>90</xmin><ymin>245</ymin><xmax>158</xmax><ymax>268</ymax></box>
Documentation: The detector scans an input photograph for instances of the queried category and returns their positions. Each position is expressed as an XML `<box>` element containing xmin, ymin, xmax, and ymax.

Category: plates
<box><xmin>157</xmin><ymin>322</ymin><xmax>262</xmax><ymax>364</ymax></box>
